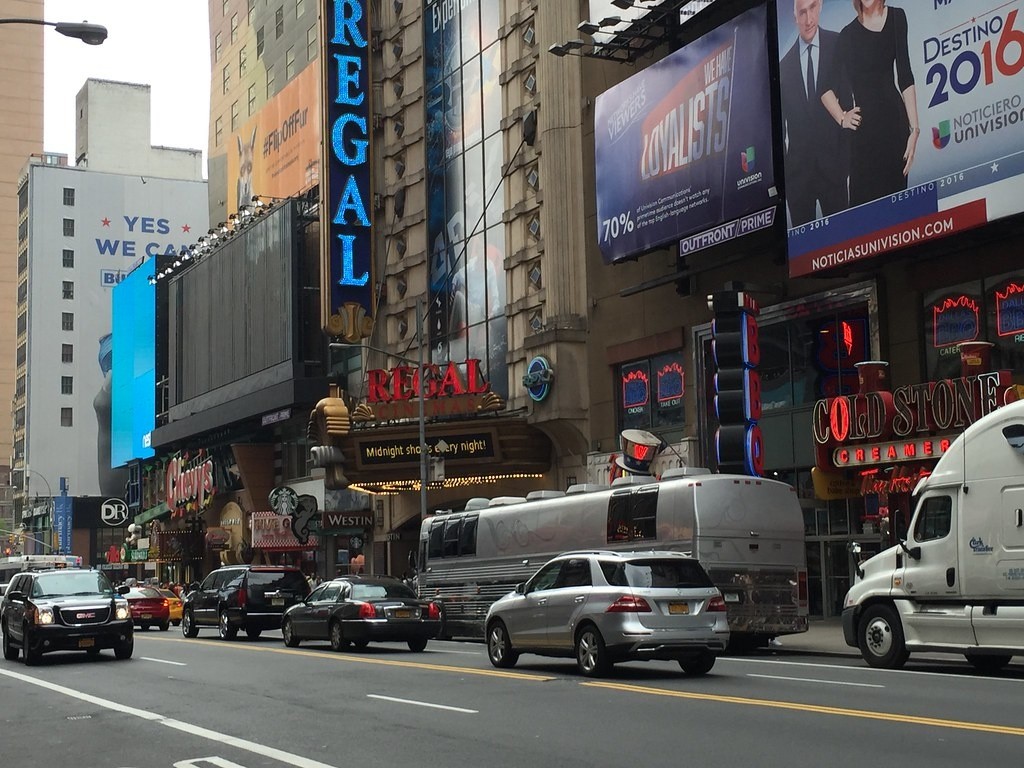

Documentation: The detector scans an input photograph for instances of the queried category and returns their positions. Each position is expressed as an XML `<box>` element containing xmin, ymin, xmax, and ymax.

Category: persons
<box><xmin>779</xmin><ymin>0</ymin><xmax>921</xmax><ymax>227</ymax></box>
<box><xmin>161</xmin><ymin>581</ymin><xmax>189</xmax><ymax>599</ymax></box>
<box><xmin>402</xmin><ymin>571</ymin><xmax>418</xmax><ymax>593</ymax></box>
<box><xmin>307</xmin><ymin>572</ymin><xmax>322</xmax><ymax>592</ymax></box>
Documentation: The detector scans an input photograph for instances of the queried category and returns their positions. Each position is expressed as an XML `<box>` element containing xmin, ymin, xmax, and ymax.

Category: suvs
<box><xmin>0</xmin><ymin>560</ymin><xmax>135</xmax><ymax>667</ymax></box>
<box><xmin>181</xmin><ymin>565</ymin><xmax>311</xmax><ymax>642</ymax></box>
<box><xmin>484</xmin><ymin>548</ymin><xmax>729</xmax><ymax>678</ymax></box>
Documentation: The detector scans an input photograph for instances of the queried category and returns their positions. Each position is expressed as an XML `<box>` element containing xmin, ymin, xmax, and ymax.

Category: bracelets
<box><xmin>840</xmin><ymin>110</ymin><xmax>845</xmax><ymax>128</ymax></box>
<box><xmin>910</xmin><ymin>127</ymin><xmax>920</xmax><ymax>134</ymax></box>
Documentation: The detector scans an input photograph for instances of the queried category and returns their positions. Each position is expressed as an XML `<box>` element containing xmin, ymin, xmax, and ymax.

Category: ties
<box><xmin>807</xmin><ymin>45</ymin><xmax>815</xmax><ymax>104</ymax></box>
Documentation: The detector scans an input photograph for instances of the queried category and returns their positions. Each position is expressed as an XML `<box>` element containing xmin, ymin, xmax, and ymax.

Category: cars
<box><xmin>159</xmin><ymin>588</ymin><xmax>184</xmax><ymax>626</ymax></box>
<box><xmin>114</xmin><ymin>577</ymin><xmax>169</xmax><ymax>631</ymax></box>
<box><xmin>281</xmin><ymin>573</ymin><xmax>442</xmax><ymax>652</ymax></box>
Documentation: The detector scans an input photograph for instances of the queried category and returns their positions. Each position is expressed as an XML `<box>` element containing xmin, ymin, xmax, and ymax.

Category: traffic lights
<box><xmin>9</xmin><ymin>534</ymin><xmax>13</xmax><ymax>544</ymax></box>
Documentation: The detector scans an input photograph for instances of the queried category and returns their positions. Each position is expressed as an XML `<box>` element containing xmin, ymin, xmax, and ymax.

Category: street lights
<box><xmin>12</xmin><ymin>468</ymin><xmax>55</xmax><ymax>555</ymax></box>
<box><xmin>328</xmin><ymin>298</ymin><xmax>429</xmax><ymax>521</ymax></box>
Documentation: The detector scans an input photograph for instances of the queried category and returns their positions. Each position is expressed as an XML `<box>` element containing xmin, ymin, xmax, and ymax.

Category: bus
<box><xmin>416</xmin><ymin>465</ymin><xmax>810</xmax><ymax>656</ymax></box>
<box><xmin>0</xmin><ymin>554</ymin><xmax>82</xmax><ymax>609</ymax></box>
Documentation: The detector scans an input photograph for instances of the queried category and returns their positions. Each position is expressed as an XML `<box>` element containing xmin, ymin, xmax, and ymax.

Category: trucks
<box><xmin>840</xmin><ymin>399</ymin><xmax>1024</xmax><ymax>670</ymax></box>
